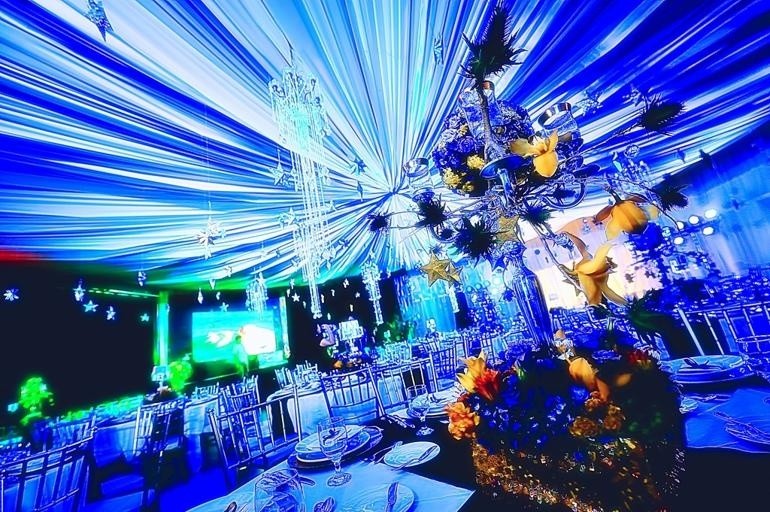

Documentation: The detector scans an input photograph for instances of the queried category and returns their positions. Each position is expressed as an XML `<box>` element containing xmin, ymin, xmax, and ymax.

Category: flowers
<box><xmin>369</xmin><ymin>1</ymin><xmax>693</xmax><ymax>336</ymax></box>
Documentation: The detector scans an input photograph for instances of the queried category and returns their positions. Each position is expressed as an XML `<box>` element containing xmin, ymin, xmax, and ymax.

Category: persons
<box><xmin>310</xmin><ymin>326</ymin><xmax>335</xmax><ymax>366</ymax></box>
<box><xmin>232</xmin><ymin>335</ymin><xmax>248</xmax><ymax>369</ymax></box>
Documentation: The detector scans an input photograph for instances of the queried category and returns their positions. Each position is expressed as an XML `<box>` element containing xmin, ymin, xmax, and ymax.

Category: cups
<box><xmin>252</xmin><ymin>467</ymin><xmax>306</xmax><ymax>512</ymax></box>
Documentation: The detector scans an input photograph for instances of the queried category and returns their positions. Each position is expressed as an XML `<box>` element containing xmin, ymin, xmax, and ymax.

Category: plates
<box><xmin>287</xmin><ymin>424</ymin><xmax>383</xmax><ymax>469</ymax></box>
<box><xmin>659</xmin><ymin>353</ymin><xmax>755</xmax><ymax>386</ymax></box>
<box><xmin>724</xmin><ymin>416</ymin><xmax>770</xmax><ymax>447</ymax></box>
<box><xmin>183</xmin><ymin>491</ymin><xmax>257</xmax><ymax>512</ymax></box>
<box><xmin>406</xmin><ymin>393</ymin><xmax>450</xmax><ymax>420</ymax></box>
<box><xmin>384</xmin><ymin>441</ymin><xmax>440</xmax><ymax>468</ymax></box>
<box><xmin>343</xmin><ymin>483</ymin><xmax>415</xmax><ymax>512</ymax></box>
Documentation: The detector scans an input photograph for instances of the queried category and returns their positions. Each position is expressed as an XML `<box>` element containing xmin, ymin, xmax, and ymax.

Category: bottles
<box><xmin>603</xmin><ymin>317</ymin><xmax>619</xmax><ymax>353</ymax></box>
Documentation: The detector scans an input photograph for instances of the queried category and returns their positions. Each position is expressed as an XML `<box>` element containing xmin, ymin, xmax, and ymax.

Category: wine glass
<box><xmin>733</xmin><ymin>334</ymin><xmax>770</xmax><ymax>406</ymax></box>
<box><xmin>406</xmin><ymin>384</ymin><xmax>435</xmax><ymax>436</ymax></box>
<box><xmin>316</xmin><ymin>417</ymin><xmax>353</xmax><ymax>487</ymax></box>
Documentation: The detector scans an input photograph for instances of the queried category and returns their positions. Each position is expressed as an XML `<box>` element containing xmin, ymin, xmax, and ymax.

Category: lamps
<box><xmin>661</xmin><ymin>207</ymin><xmax>722</xmax><ymax>246</ymax></box>
